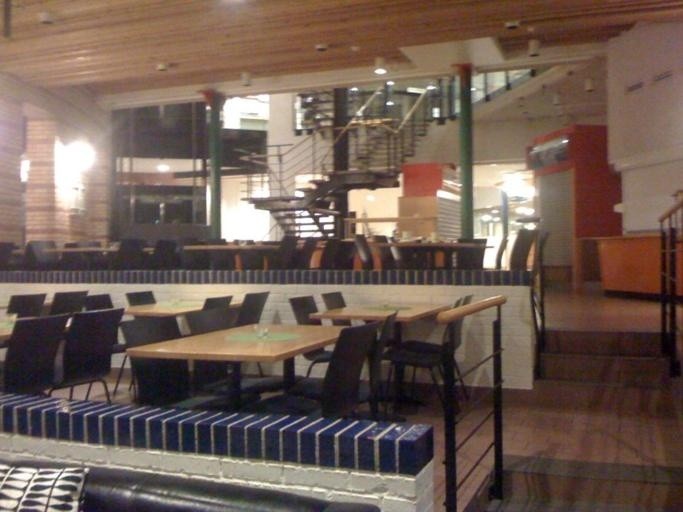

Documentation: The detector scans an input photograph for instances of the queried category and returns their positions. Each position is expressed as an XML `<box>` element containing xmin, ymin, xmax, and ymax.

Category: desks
<box><xmin>585</xmin><ymin>233</ymin><xmax>682</xmax><ymax>298</ymax></box>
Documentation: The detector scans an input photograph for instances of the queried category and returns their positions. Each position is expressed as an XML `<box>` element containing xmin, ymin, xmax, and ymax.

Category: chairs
<box><xmin>0</xmin><ymin>227</ymin><xmax>553</xmax><ymax>287</ymax></box>
<box><xmin>0</xmin><ymin>286</ymin><xmax>510</xmax><ymax>426</ymax></box>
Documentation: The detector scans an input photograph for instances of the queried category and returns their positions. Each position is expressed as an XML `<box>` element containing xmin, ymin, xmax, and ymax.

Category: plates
<box><xmin>397</xmin><ymin>236</ymin><xmax>423</xmax><ymax>244</ymax></box>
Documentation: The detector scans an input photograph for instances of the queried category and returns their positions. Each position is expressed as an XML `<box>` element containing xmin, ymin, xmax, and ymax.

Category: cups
<box><xmin>401</xmin><ymin>230</ymin><xmax>412</xmax><ymax>239</ymax></box>
<box><xmin>253</xmin><ymin>324</ymin><xmax>270</xmax><ymax>339</ymax></box>
